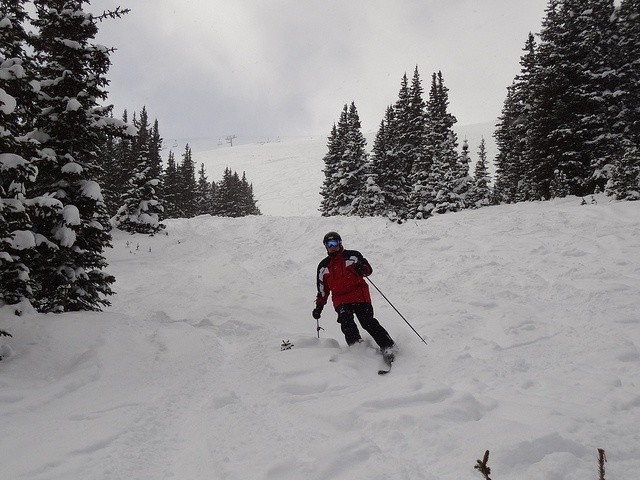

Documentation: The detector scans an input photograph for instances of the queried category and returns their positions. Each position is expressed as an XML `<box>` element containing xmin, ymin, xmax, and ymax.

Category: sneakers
<box><xmin>382</xmin><ymin>343</ymin><xmax>398</xmax><ymax>362</ymax></box>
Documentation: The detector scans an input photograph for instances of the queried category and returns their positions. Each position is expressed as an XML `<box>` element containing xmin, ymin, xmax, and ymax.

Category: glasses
<box><xmin>324</xmin><ymin>238</ymin><xmax>342</xmax><ymax>247</ymax></box>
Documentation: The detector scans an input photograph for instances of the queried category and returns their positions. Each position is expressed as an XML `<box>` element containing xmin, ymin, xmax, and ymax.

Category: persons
<box><xmin>312</xmin><ymin>232</ymin><xmax>396</xmax><ymax>363</ymax></box>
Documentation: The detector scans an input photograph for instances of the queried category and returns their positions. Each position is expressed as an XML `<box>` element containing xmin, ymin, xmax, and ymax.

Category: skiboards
<box><xmin>330</xmin><ymin>344</ymin><xmax>393</xmax><ymax>375</ymax></box>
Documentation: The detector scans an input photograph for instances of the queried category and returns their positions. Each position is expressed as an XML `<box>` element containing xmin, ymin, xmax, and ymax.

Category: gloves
<box><xmin>313</xmin><ymin>304</ymin><xmax>323</xmax><ymax>319</ymax></box>
<box><xmin>354</xmin><ymin>262</ymin><xmax>366</xmax><ymax>275</ymax></box>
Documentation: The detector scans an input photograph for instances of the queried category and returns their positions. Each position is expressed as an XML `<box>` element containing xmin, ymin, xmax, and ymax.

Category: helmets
<box><xmin>323</xmin><ymin>232</ymin><xmax>342</xmax><ymax>247</ymax></box>
